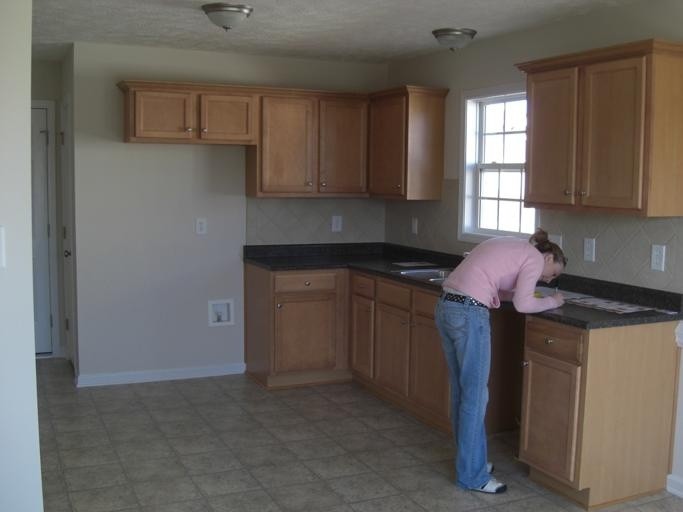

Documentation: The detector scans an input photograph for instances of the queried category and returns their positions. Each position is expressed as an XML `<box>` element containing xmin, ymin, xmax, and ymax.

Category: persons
<box><xmin>435</xmin><ymin>227</ymin><xmax>566</xmax><ymax>493</ymax></box>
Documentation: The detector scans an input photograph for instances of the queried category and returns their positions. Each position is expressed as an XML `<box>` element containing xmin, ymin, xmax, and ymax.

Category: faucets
<box><xmin>462</xmin><ymin>251</ymin><xmax>471</xmax><ymax>258</ymax></box>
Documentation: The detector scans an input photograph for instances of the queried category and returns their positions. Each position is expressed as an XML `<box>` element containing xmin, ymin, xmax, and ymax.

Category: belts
<box><xmin>438</xmin><ymin>292</ymin><xmax>492</xmax><ymax>307</ymax></box>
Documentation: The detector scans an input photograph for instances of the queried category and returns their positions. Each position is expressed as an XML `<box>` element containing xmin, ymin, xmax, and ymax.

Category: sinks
<box><xmin>399</xmin><ymin>267</ymin><xmax>456</xmax><ymax>286</ymax></box>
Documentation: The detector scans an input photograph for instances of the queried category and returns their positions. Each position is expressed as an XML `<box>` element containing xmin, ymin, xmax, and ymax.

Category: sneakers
<box><xmin>470</xmin><ymin>462</ymin><xmax>508</xmax><ymax>494</ymax></box>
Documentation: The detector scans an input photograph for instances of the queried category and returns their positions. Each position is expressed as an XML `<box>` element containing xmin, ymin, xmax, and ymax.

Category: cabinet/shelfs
<box><xmin>375</xmin><ymin>271</ymin><xmax>520</xmax><ymax>445</ymax></box>
<box><xmin>241</xmin><ymin>255</ymin><xmax>349</xmax><ymax>391</ymax></box>
<box><xmin>112</xmin><ymin>78</ymin><xmax>261</xmax><ymax>152</ymax></box>
<box><xmin>345</xmin><ymin>268</ymin><xmax>376</xmax><ymax>397</ymax></box>
<box><xmin>516</xmin><ymin>311</ymin><xmax>682</xmax><ymax>512</ymax></box>
<box><xmin>243</xmin><ymin>85</ymin><xmax>368</xmax><ymax>199</ymax></box>
<box><xmin>510</xmin><ymin>33</ymin><xmax>683</xmax><ymax>219</ymax></box>
<box><xmin>368</xmin><ymin>79</ymin><xmax>452</xmax><ymax>203</ymax></box>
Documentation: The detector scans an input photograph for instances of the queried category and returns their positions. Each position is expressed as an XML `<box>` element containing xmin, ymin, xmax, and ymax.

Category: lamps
<box><xmin>198</xmin><ymin>1</ymin><xmax>255</xmax><ymax>36</ymax></box>
<box><xmin>429</xmin><ymin>26</ymin><xmax>480</xmax><ymax>55</ymax></box>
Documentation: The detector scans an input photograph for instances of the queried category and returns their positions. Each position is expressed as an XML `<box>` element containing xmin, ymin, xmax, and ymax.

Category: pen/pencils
<box><xmin>555</xmin><ymin>286</ymin><xmax>558</xmax><ymax>293</ymax></box>
